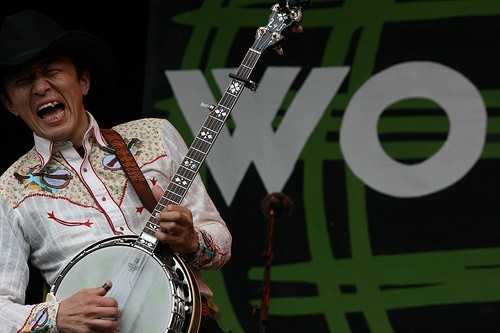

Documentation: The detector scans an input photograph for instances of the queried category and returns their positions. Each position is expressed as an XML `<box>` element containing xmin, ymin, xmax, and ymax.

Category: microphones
<box><xmin>262</xmin><ymin>190</ymin><xmax>295</xmax><ymax>222</ymax></box>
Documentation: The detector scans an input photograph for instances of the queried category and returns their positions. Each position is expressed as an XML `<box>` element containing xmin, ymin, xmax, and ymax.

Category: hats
<box><xmin>0</xmin><ymin>11</ymin><xmax>94</xmax><ymax>70</ymax></box>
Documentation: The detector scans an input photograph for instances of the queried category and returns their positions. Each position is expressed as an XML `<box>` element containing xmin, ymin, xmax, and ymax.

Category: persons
<box><xmin>1</xmin><ymin>21</ymin><xmax>235</xmax><ymax>333</ymax></box>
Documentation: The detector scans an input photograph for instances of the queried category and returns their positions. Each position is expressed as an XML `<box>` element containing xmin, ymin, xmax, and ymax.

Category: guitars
<box><xmin>48</xmin><ymin>2</ymin><xmax>302</xmax><ymax>333</ymax></box>
